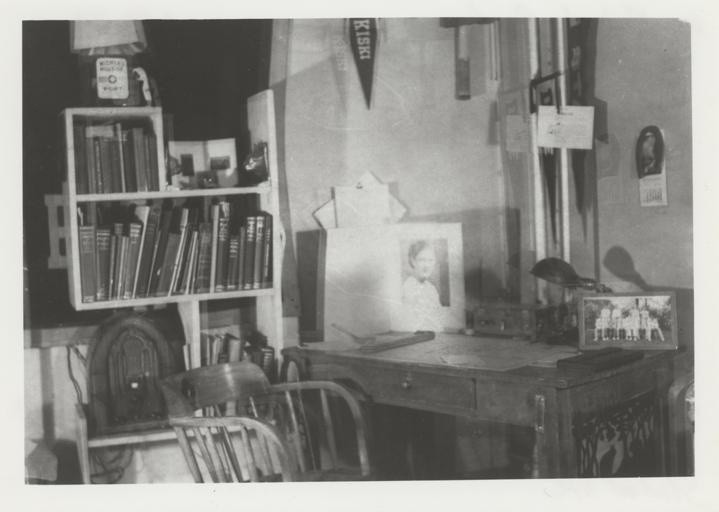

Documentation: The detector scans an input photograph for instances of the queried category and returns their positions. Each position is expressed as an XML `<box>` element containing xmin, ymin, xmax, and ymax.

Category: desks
<box><xmin>298</xmin><ymin>333</ymin><xmax>686</xmax><ymax>480</ymax></box>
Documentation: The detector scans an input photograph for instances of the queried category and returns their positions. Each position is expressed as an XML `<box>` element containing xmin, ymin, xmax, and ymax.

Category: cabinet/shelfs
<box><xmin>58</xmin><ymin>91</ymin><xmax>290</xmax><ymax>485</ymax></box>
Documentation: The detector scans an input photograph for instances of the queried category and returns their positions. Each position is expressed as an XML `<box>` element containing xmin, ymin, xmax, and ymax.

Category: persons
<box><xmin>402</xmin><ymin>240</ymin><xmax>451</xmax><ymax>310</ymax></box>
<box><xmin>592</xmin><ymin>302</ymin><xmax>666</xmax><ymax>343</ymax></box>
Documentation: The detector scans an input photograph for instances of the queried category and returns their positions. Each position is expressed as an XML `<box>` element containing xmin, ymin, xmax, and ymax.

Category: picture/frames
<box><xmin>384</xmin><ymin>222</ymin><xmax>466</xmax><ymax>332</ymax></box>
<box><xmin>578</xmin><ymin>290</ymin><xmax>679</xmax><ymax>351</ymax></box>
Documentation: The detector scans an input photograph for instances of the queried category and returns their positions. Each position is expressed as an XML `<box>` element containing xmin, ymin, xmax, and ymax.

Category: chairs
<box><xmin>162</xmin><ymin>361</ymin><xmax>371</xmax><ymax>482</ymax></box>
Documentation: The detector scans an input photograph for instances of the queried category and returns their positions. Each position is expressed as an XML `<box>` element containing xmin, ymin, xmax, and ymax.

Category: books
<box><xmin>350</xmin><ymin>327</ymin><xmax>436</xmax><ymax>354</ymax></box>
<box><xmin>555</xmin><ymin>344</ymin><xmax>646</xmax><ymax>370</ymax></box>
<box><xmin>79</xmin><ymin>198</ymin><xmax>275</xmax><ymax>306</ymax></box>
<box><xmin>72</xmin><ymin>120</ymin><xmax>159</xmax><ymax>194</ymax></box>
<box><xmin>202</xmin><ymin>327</ymin><xmax>277</xmax><ymax>424</ymax></box>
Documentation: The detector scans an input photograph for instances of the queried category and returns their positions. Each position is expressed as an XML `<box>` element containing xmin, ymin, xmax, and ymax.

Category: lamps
<box><xmin>530</xmin><ymin>258</ymin><xmax>618</xmax><ymax>294</ymax></box>
